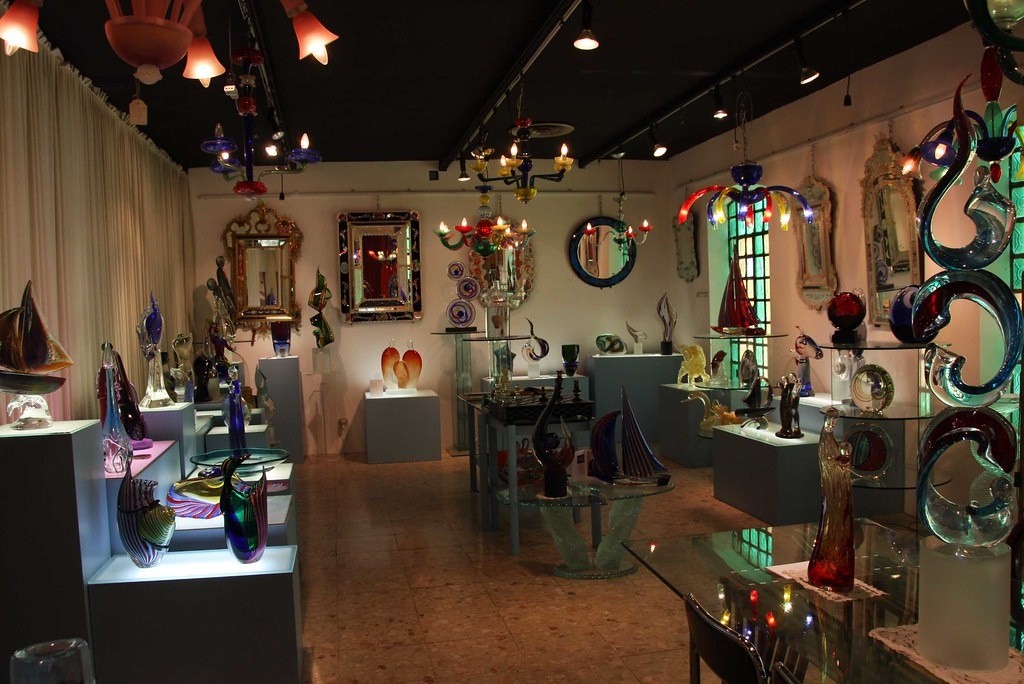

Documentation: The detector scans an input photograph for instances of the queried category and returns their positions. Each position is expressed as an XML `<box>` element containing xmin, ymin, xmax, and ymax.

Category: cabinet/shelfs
<box><xmin>818</xmin><ymin>341</ymin><xmax>952</xmax><ymax>544</ymax></box>
<box><xmin>694</xmin><ymin>332</ymin><xmax>789</xmax><ymax>439</ymax></box>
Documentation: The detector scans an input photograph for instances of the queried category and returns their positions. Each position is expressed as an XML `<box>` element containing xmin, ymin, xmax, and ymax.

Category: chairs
<box><xmin>684</xmin><ymin>592</ymin><xmax>768</xmax><ymax>684</ymax></box>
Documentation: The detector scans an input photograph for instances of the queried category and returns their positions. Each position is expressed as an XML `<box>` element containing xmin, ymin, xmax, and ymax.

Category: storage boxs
<box><xmin>482</xmin><ymin>390</ymin><xmax>596</xmax><ymax>427</ymax></box>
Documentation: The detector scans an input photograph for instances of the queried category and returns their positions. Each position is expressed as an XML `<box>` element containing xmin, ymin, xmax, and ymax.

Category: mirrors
<box><xmin>223</xmin><ymin>200</ymin><xmax>302</xmax><ymax>334</ymax></box>
<box><xmin>469</xmin><ymin>217</ymin><xmax>534</xmax><ymax>311</ymax></box>
<box><xmin>858</xmin><ymin>130</ymin><xmax>925</xmax><ymax>327</ymax></box>
<box><xmin>570</xmin><ymin>217</ymin><xmax>637</xmax><ymax>287</ymax></box>
<box><xmin>790</xmin><ymin>174</ymin><xmax>838</xmax><ymax>312</ymax></box>
<box><xmin>671</xmin><ymin>209</ymin><xmax>700</xmax><ymax>282</ymax></box>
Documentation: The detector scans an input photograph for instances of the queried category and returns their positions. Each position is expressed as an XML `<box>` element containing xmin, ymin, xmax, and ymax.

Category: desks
<box><xmin>622</xmin><ymin>519</ymin><xmax>1024</xmax><ymax>684</ymax></box>
<box><xmin>455</xmin><ymin>394</ymin><xmax>602</xmax><ymax>555</ymax></box>
<box><xmin>492</xmin><ymin>473</ymin><xmax>676</xmax><ymax>579</ymax></box>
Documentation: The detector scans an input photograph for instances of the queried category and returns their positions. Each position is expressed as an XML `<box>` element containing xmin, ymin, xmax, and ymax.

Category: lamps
<box><xmin>458</xmin><ymin>150</ymin><xmax>471</xmax><ymax>183</ymax></box>
<box><xmin>268</xmin><ymin>114</ymin><xmax>285</xmax><ymax>141</ymax></box>
<box><xmin>793</xmin><ymin>38</ymin><xmax>820</xmax><ymax>84</ymax></box>
<box><xmin>678</xmin><ymin>74</ymin><xmax>815</xmax><ymax>231</ymax></box>
<box><xmin>573</xmin><ymin>0</ymin><xmax>599</xmax><ymax>50</ymax></box>
<box><xmin>713</xmin><ymin>84</ymin><xmax>727</xmax><ymax>120</ymax></box>
<box><xmin>0</xmin><ymin>0</ymin><xmax>41</xmax><ymax>56</ymax></box>
<box><xmin>650</xmin><ymin>123</ymin><xmax>668</xmax><ymax>158</ymax></box>
<box><xmin>582</xmin><ymin>145</ymin><xmax>653</xmax><ymax>251</ymax></box>
<box><xmin>431</xmin><ymin>185</ymin><xmax>536</xmax><ymax>259</ymax></box>
<box><xmin>368</xmin><ymin>236</ymin><xmax>397</xmax><ymax>270</ymax></box>
<box><xmin>899</xmin><ymin>0</ymin><xmax>1024</xmax><ymax>186</ymax></box>
<box><xmin>200</xmin><ymin>45</ymin><xmax>322</xmax><ymax>201</ymax></box>
<box><xmin>280</xmin><ymin>0</ymin><xmax>340</xmax><ymax>66</ymax></box>
<box><xmin>182</xmin><ymin>0</ymin><xmax>227</xmax><ymax>88</ymax></box>
<box><xmin>103</xmin><ymin>0</ymin><xmax>206</xmax><ymax>87</ymax></box>
<box><xmin>470</xmin><ymin>72</ymin><xmax>574</xmax><ymax>204</ymax></box>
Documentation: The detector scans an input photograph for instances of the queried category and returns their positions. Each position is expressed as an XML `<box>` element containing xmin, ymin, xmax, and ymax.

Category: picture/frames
<box><xmin>338</xmin><ymin>210</ymin><xmax>424</xmax><ymax>327</ymax></box>
